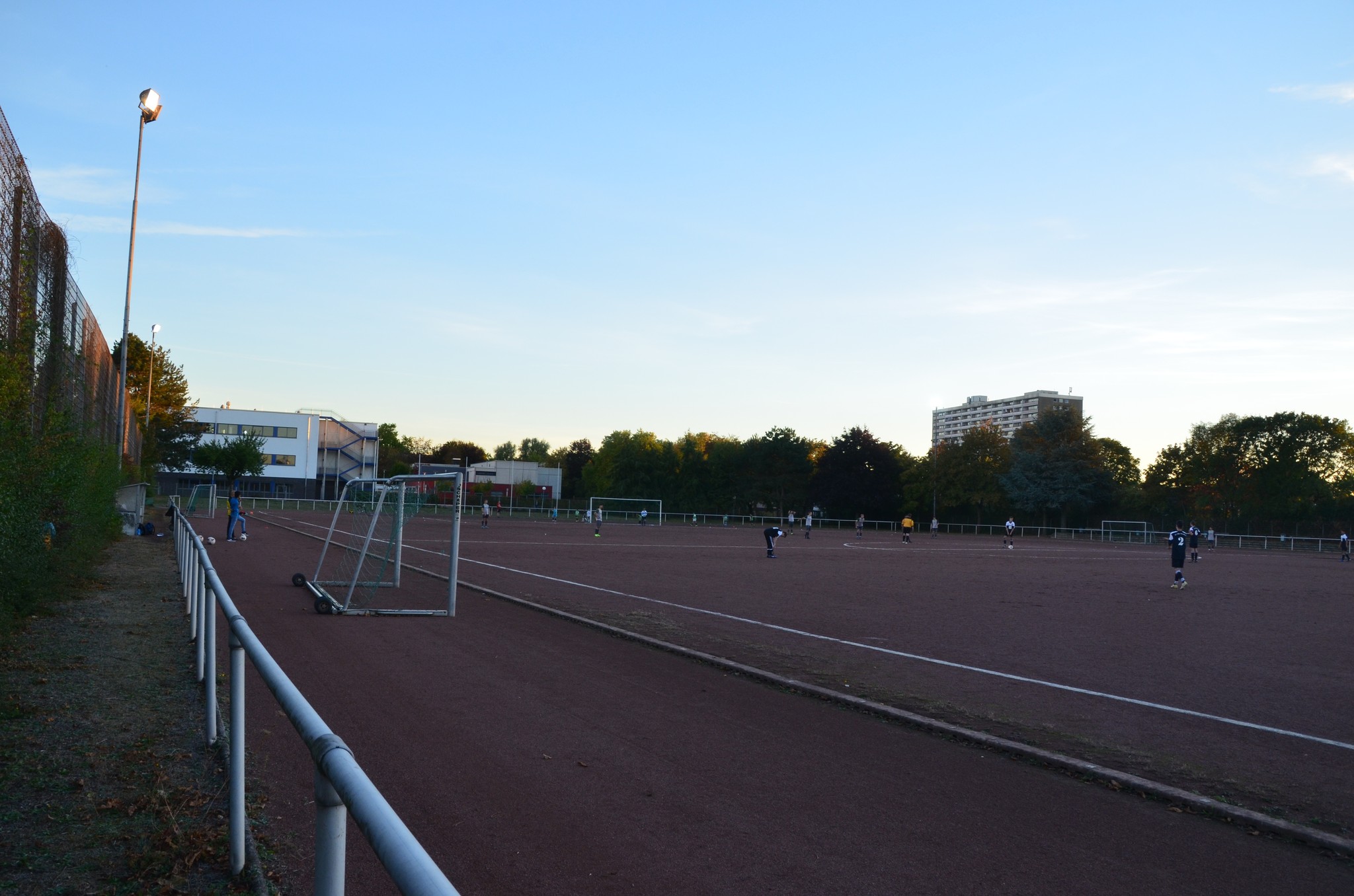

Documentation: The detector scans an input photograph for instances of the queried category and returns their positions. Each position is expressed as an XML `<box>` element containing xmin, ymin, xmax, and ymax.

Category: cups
<box><xmin>136</xmin><ymin>529</ymin><xmax>141</xmax><ymax>536</ymax></box>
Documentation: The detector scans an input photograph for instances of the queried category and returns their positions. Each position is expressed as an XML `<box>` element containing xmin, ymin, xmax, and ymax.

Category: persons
<box><xmin>1163</xmin><ymin>520</ymin><xmax>1188</xmax><ymax>590</ymax></box>
<box><xmin>481</xmin><ymin>499</ymin><xmax>491</xmax><ymax>528</ymax></box>
<box><xmin>594</xmin><ymin>504</ymin><xmax>603</xmax><ymax>537</ymax></box>
<box><xmin>638</xmin><ymin>515</ymin><xmax>642</xmax><ymax>525</ymax></box>
<box><xmin>855</xmin><ymin>514</ymin><xmax>865</xmax><ymax>539</ymax></box>
<box><xmin>551</xmin><ymin>506</ymin><xmax>557</xmax><ymax>521</ymax></box>
<box><xmin>930</xmin><ymin>516</ymin><xmax>939</xmax><ymax>539</ymax></box>
<box><xmin>1338</xmin><ymin>529</ymin><xmax>1351</xmax><ymax>562</ymax></box>
<box><xmin>575</xmin><ymin>508</ymin><xmax>590</xmax><ymax>523</ymax></box>
<box><xmin>748</xmin><ymin>513</ymin><xmax>755</xmax><ymax>528</ymax></box>
<box><xmin>641</xmin><ymin>508</ymin><xmax>647</xmax><ymax>526</ymax></box>
<box><xmin>1206</xmin><ymin>527</ymin><xmax>1215</xmax><ymax>552</ymax></box>
<box><xmin>763</xmin><ymin>527</ymin><xmax>787</xmax><ymax>558</ymax></box>
<box><xmin>497</xmin><ymin>501</ymin><xmax>501</xmax><ymax>518</ymax></box>
<box><xmin>227</xmin><ymin>491</ymin><xmax>249</xmax><ymax>542</ymax></box>
<box><xmin>1002</xmin><ymin>516</ymin><xmax>1015</xmax><ymax>549</ymax></box>
<box><xmin>690</xmin><ymin>512</ymin><xmax>698</xmax><ymax>527</ymax></box>
<box><xmin>797</xmin><ymin>511</ymin><xmax>812</xmax><ymax>539</ymax></box>
<box><xmin>901</xmin><ymin>513</ymin><xmax>914</xmax><ymax>544</ymax></box>
<box><xmin>787</xmin><ymin>510</ymin><xmax>796</xmax><ymax>535</ymax></box>
<box><xmin>1189</xmin><ymin>521</ymin><xmax>1201</xmax><ymax>562</ymax></box>
<box><xmin>722</xmin><ymin>513</ymin><xmax>728</xmax><ymax>527</ymax></box>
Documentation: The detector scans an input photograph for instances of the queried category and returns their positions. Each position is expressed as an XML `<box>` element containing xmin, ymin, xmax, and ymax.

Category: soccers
<box><xmin>207</xmin><ymin>537</ymin><xmax>215</xmax><ymax>545</ymax></box>
<box><xmin>240</xmin><ymin>535</ymin><xmax>246</xmax><ymax>541</ymax></box>
<box><xmin>198</xmin><ymin>535</ymin><xmax>203</xmax><ymax>542</ymax></box>
<box><xmin>1008</xmin><ymin>545</ymin><xmax>1013</xmax><ymax>549</ymax></box>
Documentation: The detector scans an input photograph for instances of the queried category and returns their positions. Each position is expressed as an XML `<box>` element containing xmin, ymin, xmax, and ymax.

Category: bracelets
<box><xmin>800</xmin><ymin>519</ymin><xmax>800</xmax><ymax>520</ymax></box>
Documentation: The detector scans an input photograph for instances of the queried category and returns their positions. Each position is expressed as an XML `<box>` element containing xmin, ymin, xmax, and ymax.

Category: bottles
<box><xmin>157</xmin><ymin>533</ymin><xmax>164</xmax><ymax>536</ymax></box>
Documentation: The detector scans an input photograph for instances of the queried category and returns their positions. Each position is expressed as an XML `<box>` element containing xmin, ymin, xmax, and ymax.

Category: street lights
<box><xmin>382</xmin><ymin>469</ymin><xmax>387</xmax><ymax>489</ymax></box>
<box><xmin>146</xmin><ymin>324</ymin><xmax>161</xmax><ymax>428</ymax></box>
<box><xmin>354</xmin><ymin>477</ymin><xmax>360</xmax><ymax>502</ymax></box>
<box><xmin>116</xmin><ymin>86</ymin><xmax>162</xmax><ymax>491</ymax></box>
<box><xmin>541</xmin><ymin>487</ymin><xmax>546</xmax><ymax>513</ymax></box>
